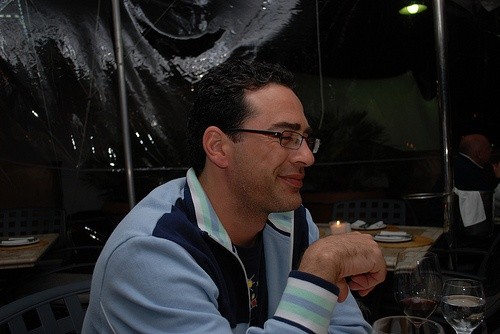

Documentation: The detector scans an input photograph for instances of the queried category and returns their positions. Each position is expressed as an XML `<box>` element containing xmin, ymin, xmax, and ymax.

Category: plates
<box><xmin>0</xmin><ymin>239</ymin><xmax>40</xmax><ymax>246</ymax></box>
<box><xmin>351</xmin><ymin>225</ymin><xmax>388</xmax><ymax>231</ymax></box>
<box><xmin>374</xmin><ymin>235</ymin><xmax>413</xmax><ymax>242</ymax></box>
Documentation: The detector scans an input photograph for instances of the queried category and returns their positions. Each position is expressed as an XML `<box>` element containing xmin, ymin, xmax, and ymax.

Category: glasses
<box><xmin>225</xmin><ymin>128</ymin><xmax>321</xmax><ymax>153</ymax></box>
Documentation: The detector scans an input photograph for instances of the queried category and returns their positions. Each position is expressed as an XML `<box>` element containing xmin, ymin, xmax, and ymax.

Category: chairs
<box><xmin>0</xmin><ymin>159</ymin><xmax>500</xmax><ymax>334</ymax></box>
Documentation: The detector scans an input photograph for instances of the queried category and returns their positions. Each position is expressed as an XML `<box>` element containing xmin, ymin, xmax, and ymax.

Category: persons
<box><xmin>448</xmin><ymin>134</ymin><xmax>500</xmax><ymax>191</ymax></box>
<box><xmin>80</xmin><ymin>57</ymin><xmax>386</xmax><ymax>334</ymax></box>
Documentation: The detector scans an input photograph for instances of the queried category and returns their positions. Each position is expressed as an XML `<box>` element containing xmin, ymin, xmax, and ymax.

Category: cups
<box><xmin>329</xmin><ymin>221</ymin><xmax>348</xmax><ymax>237</ymax></box>
<box><xmin>370</xmin><ymin>316</ymin><xmax>444</xmax><ymax>334</ymax></box>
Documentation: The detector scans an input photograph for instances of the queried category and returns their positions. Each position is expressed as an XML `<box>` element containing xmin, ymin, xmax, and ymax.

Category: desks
<box><xmin>315</xmin><ymin>222</ymin><xmax>445</xmax><ymax>326</ymax></box>
<box><xmin>0</xmin><ymin>232</ymin><xmax>60</xmax><ymax>334</ymax></box>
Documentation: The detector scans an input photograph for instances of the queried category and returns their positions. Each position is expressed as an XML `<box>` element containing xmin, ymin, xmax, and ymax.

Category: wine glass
<box><xmin>392</xmin><ymin>250</ymin><xmax>443</xmax><ymax>334</ymax></box>
<box><xmin>440</xmin><ymin>278</ymin><xmax>487</xmax><ymax>334</ymax></box>
